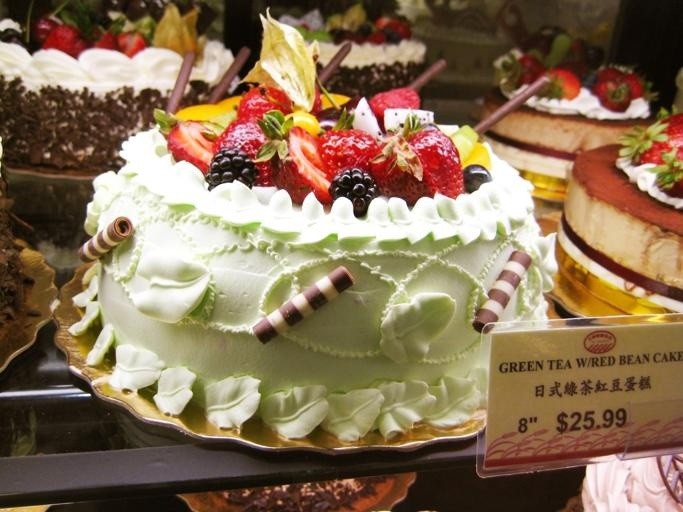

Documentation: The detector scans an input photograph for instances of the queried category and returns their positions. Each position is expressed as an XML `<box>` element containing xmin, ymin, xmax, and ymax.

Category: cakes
<box><xmin>0</xmin><ymin>0</ymin><xmax>682</xmax><ymax>511</ymax></box>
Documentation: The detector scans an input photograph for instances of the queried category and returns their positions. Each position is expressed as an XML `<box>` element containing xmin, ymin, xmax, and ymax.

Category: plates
<box><xmin>50</xmin><ymin>249</ymin><xmax>566</xmax><ymax>456</ymax></box>
<box><xmin>2</xmin><ymin>152</ymin><xmax>120</xmax><ymax>180</ymax></box>
<box><xmin>532</xmin><ymin>208</ymin><xmax>665</xmax><ymax>324</ymax></box>
<box><xmin>176</xmin><ymin>471</ymin><xmax>418</xmax><ymax>512</ymax></box>
<box><xmin>0</xmin><ymin>231</ymin><xmax>57</xmax><ymax>374</ymax></box>
